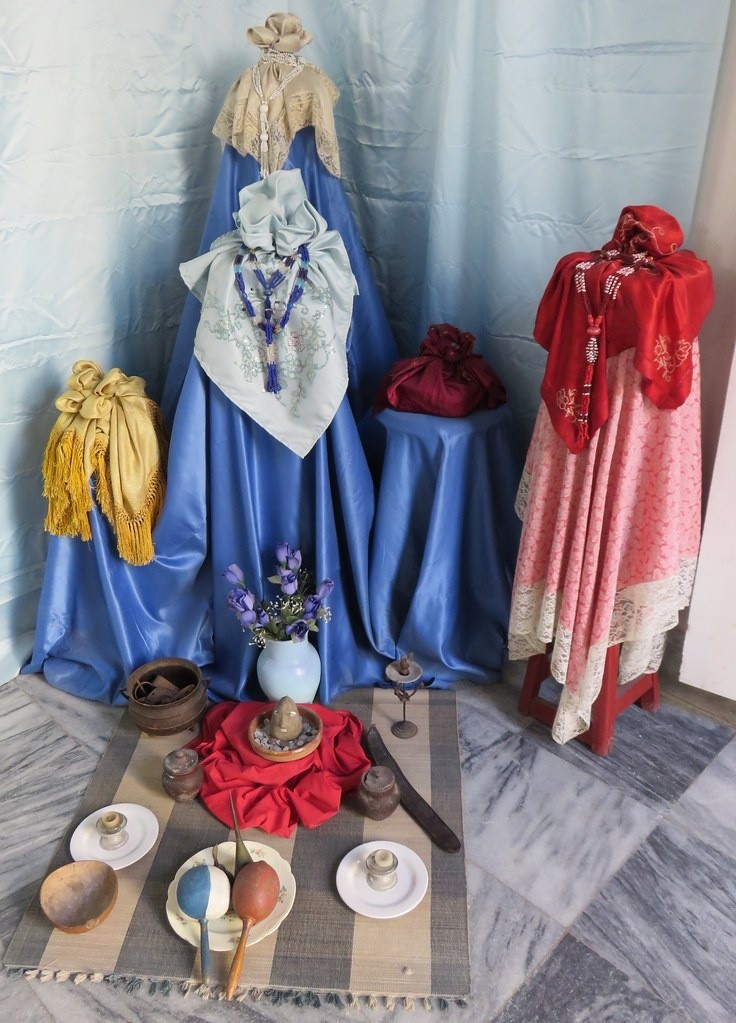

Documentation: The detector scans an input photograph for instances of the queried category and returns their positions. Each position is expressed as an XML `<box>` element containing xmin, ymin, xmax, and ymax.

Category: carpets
<box><xmin>0</xmin><ymin>688</ymin><xmax>473</xmax><ymax>1012</ymax></box>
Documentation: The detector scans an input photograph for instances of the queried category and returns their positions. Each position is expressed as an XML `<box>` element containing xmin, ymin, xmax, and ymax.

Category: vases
<box><xmin>257</xmin><ymin>635</ymin><xmax>322</xmax><ymax>705</ymax></box>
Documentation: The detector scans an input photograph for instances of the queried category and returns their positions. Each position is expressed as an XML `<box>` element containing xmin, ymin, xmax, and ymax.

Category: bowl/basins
<box><xmin>119</xmin><ymin>657</ymin><xmax>212</xmax><ymax>737</ymax></box>
<box><xmin>38</xmin><ymin>860</ymin><xmax>119</xmax><ymax>934</ymax></box>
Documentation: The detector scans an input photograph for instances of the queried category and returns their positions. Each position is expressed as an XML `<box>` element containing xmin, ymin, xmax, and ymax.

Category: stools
<box><xmin>518</xmin><ymin>638</ymin><xmax>660</xmax><ymax>754</ymax></box>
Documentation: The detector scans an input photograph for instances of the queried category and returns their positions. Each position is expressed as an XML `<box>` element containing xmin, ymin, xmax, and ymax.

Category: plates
<box><xmin>70</xmin><ymin>802</ymin><xmax>159</xmax><ymax>870</ymax></box>
<box><xmin>248</xmin><ymin>704</ymin><xmax>323</xmax><ymax>763</ymax></box>
<box><xmin>165</xmin><ymin>840</ymin><xmax>296</xmax><ymax>953</ymax></box>
<box><xmin>336</xmin><ymin>840</ymin><xmax>429</xmax><ymax>920</ymax></box>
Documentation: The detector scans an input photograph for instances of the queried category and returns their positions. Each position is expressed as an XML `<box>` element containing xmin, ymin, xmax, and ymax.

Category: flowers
<box><xmin>221</xmin><ymin>542</ymin><xmax>335</xmax><ymax>648</ymax></box>
